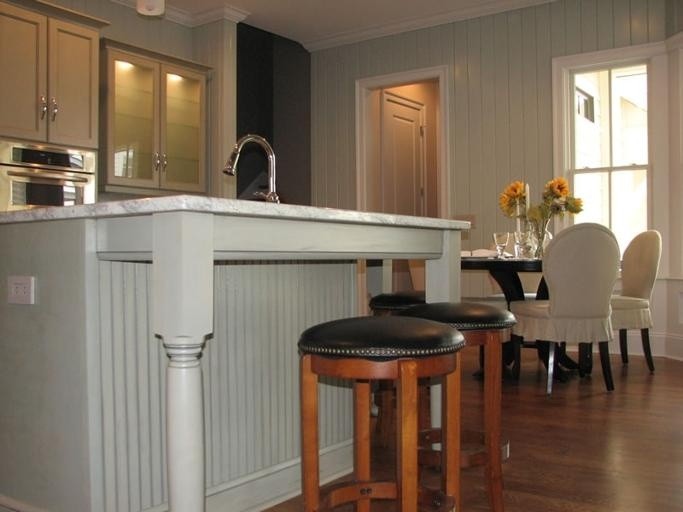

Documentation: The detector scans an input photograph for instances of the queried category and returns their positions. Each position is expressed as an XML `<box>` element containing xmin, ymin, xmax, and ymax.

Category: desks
<box><xmin>458</xmin><ymin>255</ymin><xmax>588</xmax><ymax>387</ymax></box>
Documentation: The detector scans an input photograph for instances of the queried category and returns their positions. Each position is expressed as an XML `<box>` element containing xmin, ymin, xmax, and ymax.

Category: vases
<box><xmin>527</xmin><ymin>216</ymin><xmax>548</xmax><ymax>259</ymax></box>
<box><xmin>511</xmin><ymin>203</ymin><xmax>531</xmax><ymax>256</ymax></box>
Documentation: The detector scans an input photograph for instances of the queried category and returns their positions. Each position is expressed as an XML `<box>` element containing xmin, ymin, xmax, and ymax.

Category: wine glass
<box><xmin>494</xmin><ymin>232</ymin><xmax>510</xmax><ymax>257</ymax></box>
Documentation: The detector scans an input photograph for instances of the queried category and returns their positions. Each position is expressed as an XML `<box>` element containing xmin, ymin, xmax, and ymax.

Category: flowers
<box><xmin>494</xmin><ymin>181</ymin><xmax>534</xmax><ymax>252</ymax></box>
<box><xmin>531</xmin><ymin>178</ymin><xmax>581</xmax><ymax>258</ymax></box>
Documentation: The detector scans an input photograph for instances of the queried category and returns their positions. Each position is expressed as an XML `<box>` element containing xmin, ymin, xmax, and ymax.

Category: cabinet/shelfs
<box><xmin>0</xmin><ymin>0</ymin><xmax>111</xmax><ymax>150</ymax></box>
<box><xmin>92</xmin><ymin>36</ymin><xmax>219</xmax><ymax>196</ymax></box>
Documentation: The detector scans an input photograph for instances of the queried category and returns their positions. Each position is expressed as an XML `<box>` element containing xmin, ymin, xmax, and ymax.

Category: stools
<box><xmin>407</xmin><ymin>300</ymin><xmax>519</xmax><ymax>512</ymax></box>
<box><xmin>369</xmin><ymin>289</ymin><xmax>429</xmax><ymax>423</ymax></box>
<box><xmin>296</xmin><ymin>314</ymin><xmax>467</xmax><ymax>512</ymax></box>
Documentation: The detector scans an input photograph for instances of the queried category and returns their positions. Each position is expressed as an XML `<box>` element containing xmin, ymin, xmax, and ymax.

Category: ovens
<box><xmin>0</xmin><ymin>139</ymin><xmax>95</xmax><ymax>210</ymax></box>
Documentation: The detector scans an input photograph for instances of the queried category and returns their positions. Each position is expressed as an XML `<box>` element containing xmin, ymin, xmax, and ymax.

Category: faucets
<box><xmin>222</xmin><ymin>132</ymin><xmax>281</xmax><ymax>205</ymax></box>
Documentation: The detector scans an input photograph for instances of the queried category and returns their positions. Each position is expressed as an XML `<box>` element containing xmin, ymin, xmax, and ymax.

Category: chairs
<box><xmin>512</xmin><ymin>226</ymin><xmax>617</xmax><ymax>395</ymax></box>
<box><xmin>460</xmin><ymin>248</ymin><xmax>513</xmax><ymax>310</ymax></box>
<box><xmin>607</xmin><ymin>227</ymin><xmax>659</xmax><ymax>368</ymax></box>
